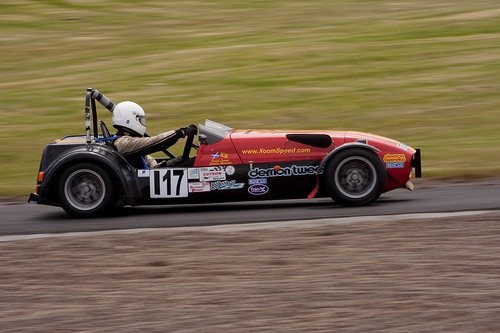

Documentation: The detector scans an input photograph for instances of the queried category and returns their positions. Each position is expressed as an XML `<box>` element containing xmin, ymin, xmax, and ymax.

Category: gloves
<box><xmin>180</xmin><ymin>124</ymin><xmax>197</xmax><ymax>138</ymax></box>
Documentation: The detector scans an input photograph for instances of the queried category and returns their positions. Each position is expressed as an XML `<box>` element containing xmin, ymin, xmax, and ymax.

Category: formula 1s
<box><xmin>26</xmin><ymin>88</ymin><xmax>422</xmax><ymax>219</ymax></box>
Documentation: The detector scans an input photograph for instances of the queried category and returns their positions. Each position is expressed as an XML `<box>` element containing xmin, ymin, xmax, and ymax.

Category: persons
<box><xmin>109</xmin><ymin>101</ymin><xmax>198</xmax><ymax>167</ymax></box>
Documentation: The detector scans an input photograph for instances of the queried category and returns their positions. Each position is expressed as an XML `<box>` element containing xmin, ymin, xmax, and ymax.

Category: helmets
<box><xmin>112</xmin><ymin>101</ymin><xmax>146</xmax><ymax>137</ymax></box>
